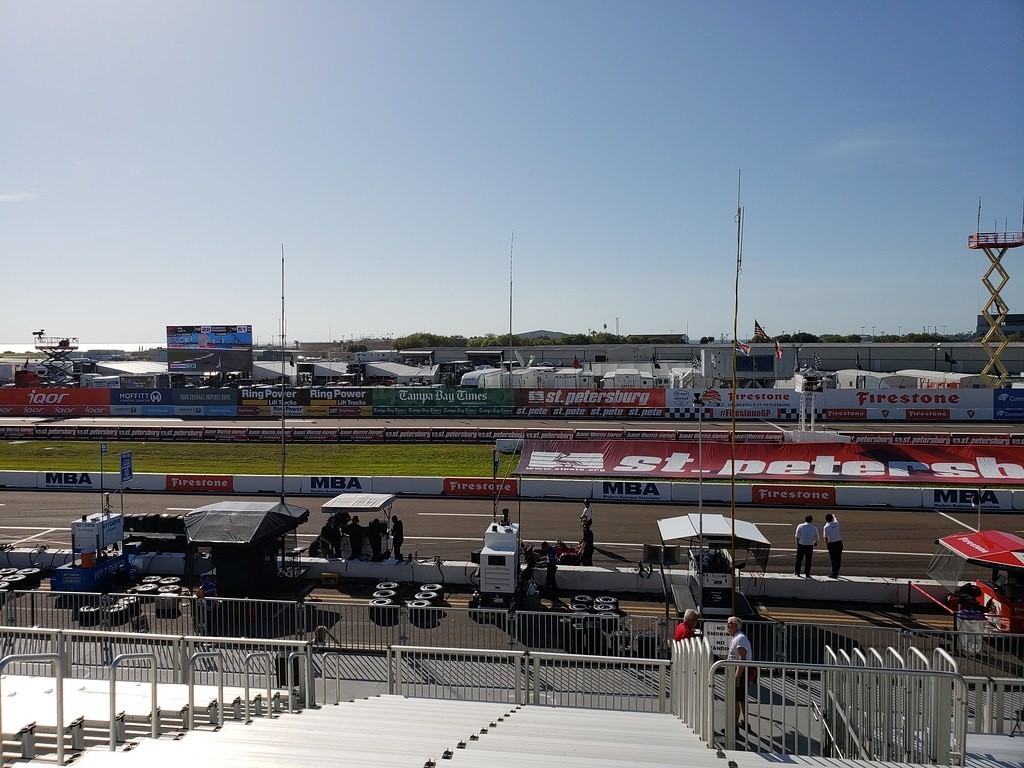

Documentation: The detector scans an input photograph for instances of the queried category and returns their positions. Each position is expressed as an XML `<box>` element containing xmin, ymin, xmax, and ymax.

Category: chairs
<box><xmin>1012</xmin><ymin>705</ymin><xmax>1024</xmax><ymax>735</ymax></box>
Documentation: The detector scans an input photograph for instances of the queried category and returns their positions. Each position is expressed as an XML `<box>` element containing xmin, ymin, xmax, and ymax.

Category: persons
<box><xmin>198</xmin><ymin>572</ymin><xmax>217</xmax><ymax>636</ymax></box>
<box><xmin>673</xmin><ymin>608</ymin><xmax>698</xmax><ymax>645</ymax></box>
<box><xmin>539</xmin><ymin>541</ymin><xmax>558</xmax><ymax>596</ymax></box>
<box><xmin>823</xmin><ymin>513</ymin><xmax>843</xmax><ymax>579</ymax></box>
<box><xmin>793</xmin><ymin>514</ymin><xmax>820</xmax><ymax>579</ymax></box>
<box><xmin>799</xmin><ymin>363</ymin><xmax>818</xmax><ymax>377</ymax></box>
<box><xmin>577</xmin><ymin>498</ymin><xmax>594</xmax><ymax>567</ymax></box>
<box><xmin>308</xmin><ymin>513</ymin><xmax>404</xmax><ymax>562</ymax></box>
<box><xmin>721</xmin><ymin>617</ymin><xmax>751</xmax><ymax>739</ymax></box>
<box><xmin>448</xmin><ymin>374</ymin><xmax>455</xmax><ymax>386</ymax></box>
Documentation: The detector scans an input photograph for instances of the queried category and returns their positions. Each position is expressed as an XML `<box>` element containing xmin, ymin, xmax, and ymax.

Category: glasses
<box><xmin>727</xmin><ymin>622</ymin><xmax>736</xmax><ymax>626</ymax></box>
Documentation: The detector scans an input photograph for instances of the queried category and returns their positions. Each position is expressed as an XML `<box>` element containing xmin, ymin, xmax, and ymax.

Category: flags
<box><xmin>571</xmin><ymin>352</ymin><xmax>581</xmax><ymax>371</ymax></box>
<box><xmin>813</xmin><ymin>351</ymin><xmax>822</xmax><ymax>371</ymax></box>
<box><xmin>651</xmin><ymin>353</ymin><xmax>662</xmax><ymax>370</ymax></box>
<box><xmin>754</xmin><ymin>318</ymin><xmax>771</xmax><ymax>344</ymax></box>
<box><xmin>735</xmin><ymin>339</ymin><xmax>750</xmax><ymax>357</ymax></box>
<box><xmin>710</xmin><ymin>352</ymin><xmax>718</xmax><ymax>370</ymax></box>
<box><xmin>944</xmin><ymin>351</ymin><xmax>958</xmax><ymax>365</ymax></box>
<box><xmin>691</xmin><ymin>354</ymin><xmax>699</xmax><ymax>370</ymax></box>
<box><xmin>856</xmin><ymin>351</ymin><xmax>863</xmax><ymax>370</ymax></box>
<box><xmin>773</xmin><ymin>338</ymin><xmax>783</xmax><ymax>361</ymax></box>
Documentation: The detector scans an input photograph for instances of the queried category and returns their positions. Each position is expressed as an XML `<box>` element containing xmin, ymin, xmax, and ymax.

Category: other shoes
<box><xmin>721</xmin><ymin>726</ymin><xmax>739</xmax><ymax>734</ymax></box>
<box><xmin>739</xmin><ymin>720</ymin><xmax>750</xmax><ymax>729</ymax></box>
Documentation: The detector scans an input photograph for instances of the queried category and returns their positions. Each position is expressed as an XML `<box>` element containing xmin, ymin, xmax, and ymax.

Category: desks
<box><xmin>976</xmin><ymin>579</ymin><xmax>1024</xmax><ymax>634</ymax></box>
<box><xmin>955</xmin><ymin>611</ymin><xmax>988</xmax><ymax>655</ymax></box>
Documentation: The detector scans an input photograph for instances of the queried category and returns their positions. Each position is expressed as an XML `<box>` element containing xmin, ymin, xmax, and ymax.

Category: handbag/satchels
<box><xmin>748</xmin><ymin>666</ymin><xmax>757</xmax><ymax>683</ymax></box>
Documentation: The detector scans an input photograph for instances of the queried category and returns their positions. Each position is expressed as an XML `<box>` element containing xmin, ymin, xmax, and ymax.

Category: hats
<box><xmin>200</xmin><ymin>573</ymin><xmax>207</xmax><ymax>579</ymax></box>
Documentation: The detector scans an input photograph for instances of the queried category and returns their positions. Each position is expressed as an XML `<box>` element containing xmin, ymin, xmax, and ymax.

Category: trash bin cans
<box><xmin>956</xmin><ymin>609</ymin><xmax>988</xmax><ymax>654</ymax></box>
<box><xmin>636</xmin><ymin>631</ymin><xmax>660</xmax><ymax>668</ymax></box>
<box><xmin>611</xmin><ymin>630</ymin><xmax>634</xmax><ymax>668</ymax></box>
